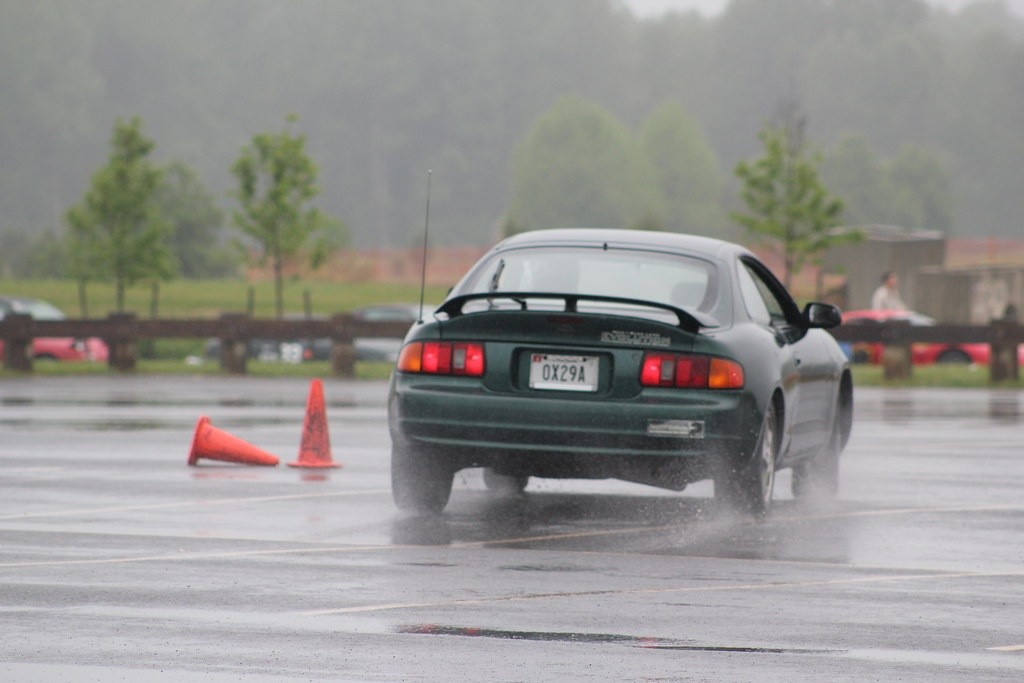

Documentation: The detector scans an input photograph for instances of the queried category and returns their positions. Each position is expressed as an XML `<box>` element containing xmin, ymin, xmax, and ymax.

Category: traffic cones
<box><xmin>186</xmin><ymin>416</ymin><xmax>280</xmax><ymax>467</ymax></box>
<box><xmin>286</xmin><ymin>379</ymin><xmax>342</xmax><ymax>469</ymax></box>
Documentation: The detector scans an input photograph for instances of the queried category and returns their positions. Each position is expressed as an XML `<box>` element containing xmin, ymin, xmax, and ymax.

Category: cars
<box><xmin>389</xmin><ymin>224</ymin><xmax>852</xmax><ymax>528</ymax></box>
<box><xmin>188</xmin><ymin>303</ymin><xmax>433</xmax><ymax>371</ymax></box>
<box><xmin>837</xmin><ymin>308</ymin><xmax>1024</xmax><ymax>369</ymax></box>
<box><xmin>0</xmin><ymin>298</ymin><xmax>108</xmax><ymax>365</ymax></box>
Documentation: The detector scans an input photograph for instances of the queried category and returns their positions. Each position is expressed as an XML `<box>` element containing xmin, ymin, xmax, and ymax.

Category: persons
<box><xmin>871</xmin><ymin>271</ymin><xmax>908</xmax><ymax>310</ymax></box>
